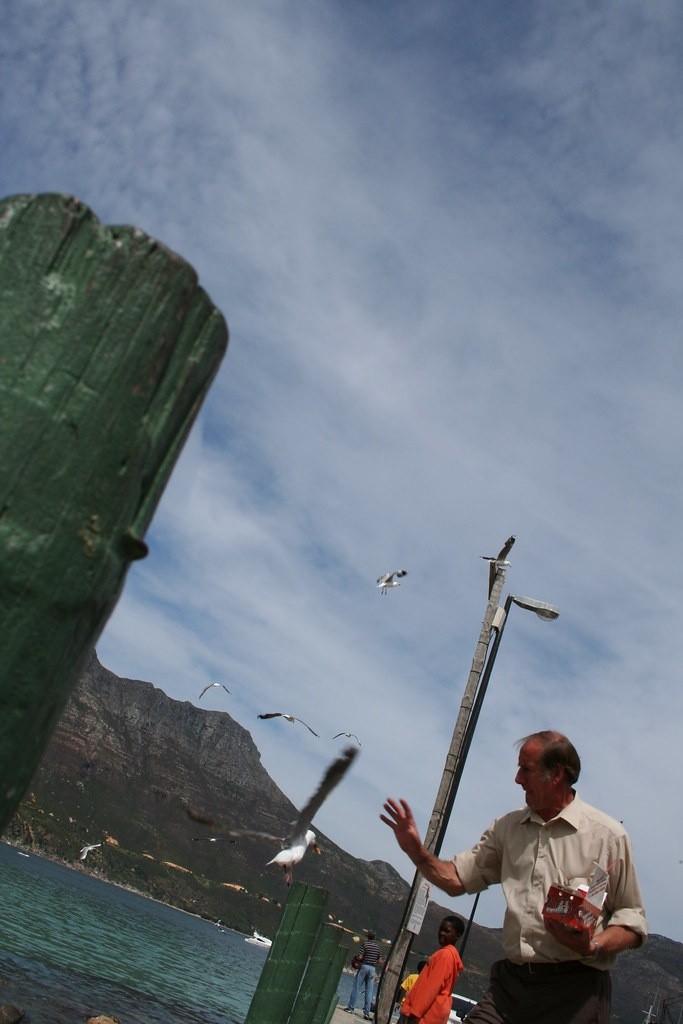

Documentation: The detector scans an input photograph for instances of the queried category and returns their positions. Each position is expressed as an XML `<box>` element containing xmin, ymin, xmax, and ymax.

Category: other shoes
<box><xmin>344</xmin><ymin>1008</ymin><xmax>353</xmax><ymax>1013</ymax></box>
<box><xmin>364</xmin><ymin>1014</ymin><xmax>369</xmax><ymax>1019</ymax></box>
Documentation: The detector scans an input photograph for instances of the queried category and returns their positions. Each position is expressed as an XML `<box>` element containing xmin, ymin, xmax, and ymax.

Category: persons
<box><xmin>396</xmin><ymin>961</ymin><xmax>428</xmax><ymax>1009</ymax></box>
<box><xmin>380</xmin><ymin>730</ymin><xmax>647</xmax><ymax>1024</ymax></box>
<box><xmin>395</xmin><ymin>916</ymin><xmax>465</xmax><ymax>1024</ymax></box>
<box><xmin>344</xmin><ymin>929</ymin><xmax>384</xmax><ymax>1020</ymax></box>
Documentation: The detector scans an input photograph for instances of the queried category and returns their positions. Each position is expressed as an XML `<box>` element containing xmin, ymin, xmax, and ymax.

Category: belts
<box><xmin>521</xmin><ymin>960</ymin><xmax>586</xmax><ymax>975</ymax></box>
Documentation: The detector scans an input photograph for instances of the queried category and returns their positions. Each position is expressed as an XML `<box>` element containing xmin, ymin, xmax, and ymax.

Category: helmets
<box><xmin>352</xmin><ymin>959</ymin><xmax>360</xmax><ymax>969</ymax></box>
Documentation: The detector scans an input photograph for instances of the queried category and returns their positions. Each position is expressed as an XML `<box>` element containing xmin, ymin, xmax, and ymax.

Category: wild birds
<box><xmin>377</xmin><ymin>569</ymin><xmax>407</xmax><ymax>596</ymax></box>
<box><xmin>213</xmin><ymin>919</ymin><xmax>226</xmax><ymax>935</ymax></box>
<box><xmin>79</xmin><ymin>843</ymin><xmax>102</xmax><ymax>861</ymax></box>
<box><xmin>187</xmin><ymin>682</ymin><xmax>361</xmax><ymax>886</ymax></box>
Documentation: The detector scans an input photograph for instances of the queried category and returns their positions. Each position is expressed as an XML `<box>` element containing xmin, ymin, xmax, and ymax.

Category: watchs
<box><xmin>578</xmin><ymin>940</ymin><xmax>599</xmax><ymax>960</ymax></box>
